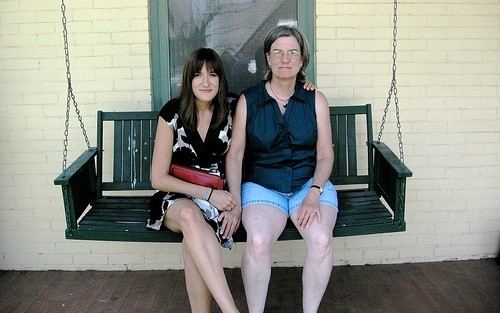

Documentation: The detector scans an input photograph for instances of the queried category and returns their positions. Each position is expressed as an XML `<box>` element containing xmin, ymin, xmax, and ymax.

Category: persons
<box><xmin>146</xmin><ymin>48</ymin><xmax>318</xmax><ymax>313</ymax></box>
<box><xmin>219</xmin><ymin>24</ymin><xmax>339</xmax><ymax>313</ymax></box>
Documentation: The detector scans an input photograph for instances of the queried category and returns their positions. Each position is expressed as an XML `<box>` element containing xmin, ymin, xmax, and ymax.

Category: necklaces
<box><xmin>269</xmin><ymin>81</ymin><xmax>295</xmax><ymax>107</ymax></box>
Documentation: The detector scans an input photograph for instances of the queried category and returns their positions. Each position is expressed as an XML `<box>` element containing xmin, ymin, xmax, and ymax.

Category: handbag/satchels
<box><xmin>169</xmin><ymin>164</ymin><xmax>224</xmax><ymax>190</ymax></box>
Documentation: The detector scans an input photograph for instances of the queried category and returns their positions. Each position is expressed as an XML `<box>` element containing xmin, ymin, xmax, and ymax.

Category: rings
<box><xmin>230</xmin><ymin>204</ymin><xmax>233</xmax><ymax>207</ymax></box>
<box><xmin>313</xmin><ymin>212</ymin><xmax>318</xmax><ymax>216</ymax></box>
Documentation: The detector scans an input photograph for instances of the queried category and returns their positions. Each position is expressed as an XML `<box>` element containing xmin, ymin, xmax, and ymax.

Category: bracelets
<box><xmin>204</xmin><ymin>187</ymin><xmax>214</xmax><ymax>201</ymax></box>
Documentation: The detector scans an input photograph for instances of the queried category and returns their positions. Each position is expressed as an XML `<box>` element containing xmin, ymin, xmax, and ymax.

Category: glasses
<box><xmin>273</xmin><ymin>49</ymin><xmax>303</xmax><ymax>58</ymax></box>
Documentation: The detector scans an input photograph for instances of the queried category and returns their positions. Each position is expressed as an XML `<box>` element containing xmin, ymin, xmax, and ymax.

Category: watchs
<box><xmin>311</xmin><ymin>184</ymin><xmax>324</xmax><ymax>196</ymax></box>
